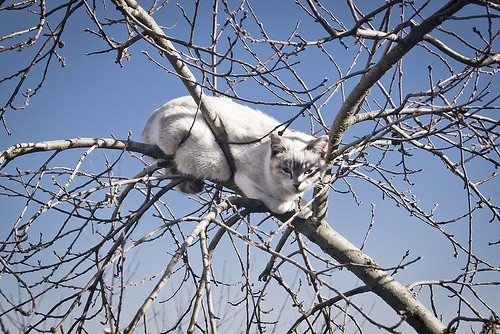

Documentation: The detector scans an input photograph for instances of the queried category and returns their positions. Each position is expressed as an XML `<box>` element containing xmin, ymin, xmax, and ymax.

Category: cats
<box><xmin>139</xmin><ymin>95</ymin><xmax>330</xmax><ymax>219</ymax></box>
